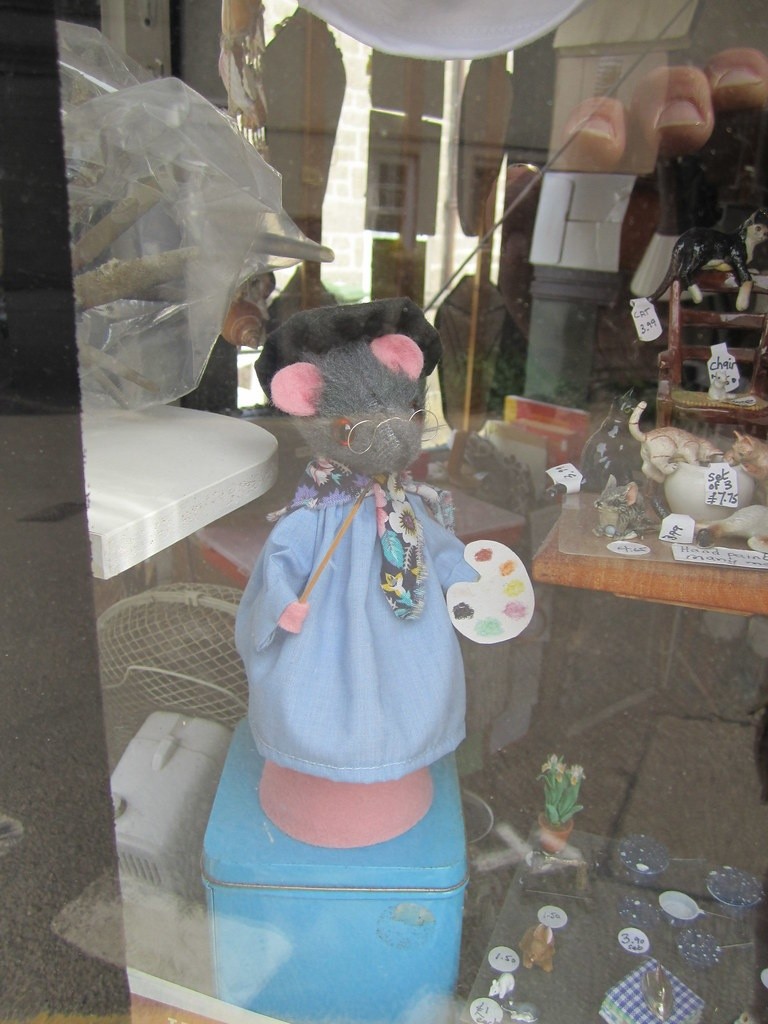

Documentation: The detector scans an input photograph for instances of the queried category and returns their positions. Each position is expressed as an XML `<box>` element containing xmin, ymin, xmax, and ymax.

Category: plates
<box><xmin>618</xmin><ymin>896</ymin><xmax>659</xmax><ymax>928</ymax></box>
<box><xmin>617</xmin><ymin>834</ymin><xmax>670</xmax><ymax>874</ymax></box>
<box><xmin>706</xmin><ymin>866</ymin><xmax>764</xmax><ymax>906</ymax></box>
<box><xmin>676</xmin><ymin>929</ymin><xmax>720</xmax><ymax>966</ymax></box>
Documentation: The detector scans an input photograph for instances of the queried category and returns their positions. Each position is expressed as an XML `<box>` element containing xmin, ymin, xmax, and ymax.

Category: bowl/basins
<box><xmin>658</xmin><ymin>891</ymin><xmax>705</xmax><ymax>927</ymax></box>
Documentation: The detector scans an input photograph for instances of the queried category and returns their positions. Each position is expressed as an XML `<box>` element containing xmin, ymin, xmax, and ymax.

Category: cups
<box><xmin>664</xmin><ymin>457</ymin><xmax>754</xmax><ymax>535</ymax></box>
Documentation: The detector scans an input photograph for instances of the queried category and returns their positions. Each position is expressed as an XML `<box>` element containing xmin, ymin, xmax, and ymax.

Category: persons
<box><xmin>479</xmin><ymin>46</ymin><xmax>768</xmax><ymax>398</ymax></box>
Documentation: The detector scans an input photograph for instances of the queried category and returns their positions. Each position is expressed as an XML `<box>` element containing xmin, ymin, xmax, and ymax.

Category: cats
<box><xmin>628</xmin><ymin>401</ymin><xmax>768</xmax><ymax>506</ymax></box>
<box><xmin>646</xmin><ymin>209</ymin><xmax>768</xmax><ymax>312</ymax></box>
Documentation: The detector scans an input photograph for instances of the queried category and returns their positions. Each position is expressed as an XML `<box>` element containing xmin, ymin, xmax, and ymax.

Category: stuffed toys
<box><xmin>233</xmin><ymin>294</ymin><xmax>479</xmax><ymax>784</ymax></box>
